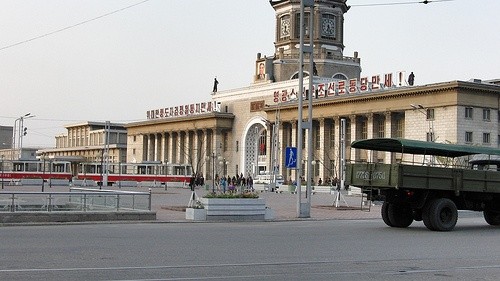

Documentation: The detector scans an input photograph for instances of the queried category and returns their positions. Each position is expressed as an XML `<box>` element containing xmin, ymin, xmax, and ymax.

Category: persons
<box><xmin>192</xmin><ymin>172</ymin><xmax>205</xmax><ymax>187</ymax></box>
<box><xmin>212</xmin><ymin>78</ymin><xmax>219</xmax><ymax>92</ymax></box>
<box><xmin>256</xmin><ymin>62</ymin><xmax>265</xmax><ymax>80</ymax></box>
<box><xmin>408</xmin><ymin>71</ymin><xmax>415</xmax><ymax>86</ymax></box>
<box><xmin>190</xmin><ymin>175</ymin><xmax>195</xmax><ymax>192</ymax></box>
<box><xmin>288</xmin><ymin>175</ymin><xmax>349</xmax><ymax>192</ymax></box>
<box><xmin>215</xmin><ymin>173</ymin><xmax>255</xmax><ymax>193</ymax></box>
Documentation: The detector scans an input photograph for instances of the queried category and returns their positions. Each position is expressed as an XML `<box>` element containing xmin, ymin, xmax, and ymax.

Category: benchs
<box><xmin>275</xmin><ymin>184</ymin><xmax>297</xmax><ymax>194</ymax></box>
<box><xmin>309</xmin><ymin>185</ymin><xmax>332</xmax><ymax>196</ymax></box>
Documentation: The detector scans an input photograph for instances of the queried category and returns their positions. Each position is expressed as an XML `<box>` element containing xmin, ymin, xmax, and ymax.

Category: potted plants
<box><xmin>254</xmin><ymin>181</ymin><xmax>268</xmax><ymax>191</ymax></box>
<box><xmin>186</xmin><ymin>200</ymin><xmax>206</xmax><ymax>221</ymax></box>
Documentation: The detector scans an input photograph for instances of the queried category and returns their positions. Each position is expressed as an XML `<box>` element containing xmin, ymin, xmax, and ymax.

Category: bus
<box><xmin>0</xmin><ymin>160</ymin><xmax>73</xmax><ymax>179</ymax></box>
<box><xmin>79</xmin><ymin>162</ymin><xmax>196</xmax><ymax>187</ymax></box>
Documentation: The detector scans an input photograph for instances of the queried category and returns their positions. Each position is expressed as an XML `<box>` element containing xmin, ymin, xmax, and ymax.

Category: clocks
<box><xmin>280</xmin><ymin>18</ymin><xmax>291</xmax><ymax>38</ymax></box>
<box><xmin>321</xmin><ymin>17</ymin><xmax>336</xmax><ymax>38</ymax></box>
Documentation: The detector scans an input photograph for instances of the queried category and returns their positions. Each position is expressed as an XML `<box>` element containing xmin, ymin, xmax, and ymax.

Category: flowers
<box><xmin>204</xmin><ymin>184</ymin><xmax>259</xmax><ymax>198</ymax></box>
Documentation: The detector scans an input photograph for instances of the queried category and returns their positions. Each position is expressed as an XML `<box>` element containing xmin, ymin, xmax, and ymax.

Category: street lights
<box><xmin>211</xmin><ymin>152</ymin><xmax>216</xmax><ymax>194</ymax></box>
<box><xmin>1</xmin><ymin>155</ymin><xmax>5</xmax><ymax>189</ymax></box>
<box><xmin>99</xmin><ymin>148</ymin><xmax>105</xmax><ymax>196</ymax></box>
<box><xmin>48</xmin><ymin>159</ymin><xmax>53</xmax><ymax>188</ymax></box>
<box><xmin>40</xmin><ymin>154</ymin><xmax>49</xmax><ymax>192</ymax></box>
<box><xmin>219</xmin><ymin>158</ymin><xmax>229</xmax><ymax>194</ymax></box>
<box><xmin>14</xmin><ymin>113</ymin><xmax>30</xmax><ymax>148</ymax></box>
<box><xmin>18</xmin><ymin>115</ymin><xmax>33</xmax><ymax>147</ymax></box>
<box><xmin>165</xmin><ymin>159</ymin><xmax>167</xmax><ymax>190</ymax></box>
<box><xmin>410</xmin><ymin>104</ymin><xmax>434</xmax><ymax>143</ymax></box>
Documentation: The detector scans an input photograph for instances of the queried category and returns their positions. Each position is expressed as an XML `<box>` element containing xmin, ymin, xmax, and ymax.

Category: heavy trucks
<box><xmin>344</xmin><ymin>138</ymin><xmax>500</xmax><ymax>231</ymax></box>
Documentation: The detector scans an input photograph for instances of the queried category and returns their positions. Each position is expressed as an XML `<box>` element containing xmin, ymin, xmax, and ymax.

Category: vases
<box><xmin>200</xmin><ymin>197</ymin><xmax>266</xmax><ymax>215</ymax></box>
